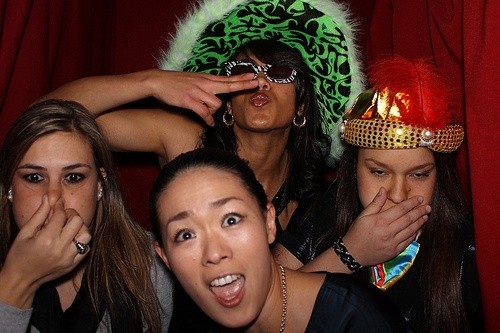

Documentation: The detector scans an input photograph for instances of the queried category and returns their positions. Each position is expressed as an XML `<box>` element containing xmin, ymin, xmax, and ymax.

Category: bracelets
<box><xmin>333</xmin><ymin>236</ymin><xmax>363</xmax><ymax>272</ymax></box>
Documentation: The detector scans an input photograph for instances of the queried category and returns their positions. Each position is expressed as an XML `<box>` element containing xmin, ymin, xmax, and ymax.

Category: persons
<box><xmin>274</xmin><ymin>56</ymin><xmax>485</xmax><ymax>333</ymax></box>
<box><xmin>0</xmin><ymin>98</ymin><xmax>174</xmax><ymax>333</ymax></box>
<box><xmin>148</xmin><ymin>147</ymin><xmax>409</xmax><ymax>333</ymax></box>
<box><xmin>29</xmin><ymin>0</ymin><xmax>365</xmax><ymax>251</ymax></box>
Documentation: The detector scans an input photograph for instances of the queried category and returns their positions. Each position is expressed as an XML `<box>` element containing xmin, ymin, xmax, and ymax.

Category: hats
<box><xmin>338</xmin><ymin>54</ymin><xmax>465</xmax><ymax>153</ymax></box>
<box><xmin>159</xmin><ymin>0</ymin><xmax>366</xmax><ymax>166</ymax></box>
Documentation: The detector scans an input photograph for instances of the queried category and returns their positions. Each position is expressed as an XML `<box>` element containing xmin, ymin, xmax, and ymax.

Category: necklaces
<box><xmin>279</xmin><ymin>264</ymin><xmax>286</xmax><ymax>333</ymax></box>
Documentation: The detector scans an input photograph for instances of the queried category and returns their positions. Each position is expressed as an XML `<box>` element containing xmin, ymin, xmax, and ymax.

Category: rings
<box><xmin>73</xmin><ymin>239</ymin><xmax>88</xmax><ymax>254</ymax></box>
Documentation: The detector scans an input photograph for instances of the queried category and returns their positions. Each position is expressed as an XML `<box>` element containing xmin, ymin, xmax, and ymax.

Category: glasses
<box><xmin>219</xmin><ymin>59</ymin><xmax>298</xmax><ymax>83</ymax></box>
